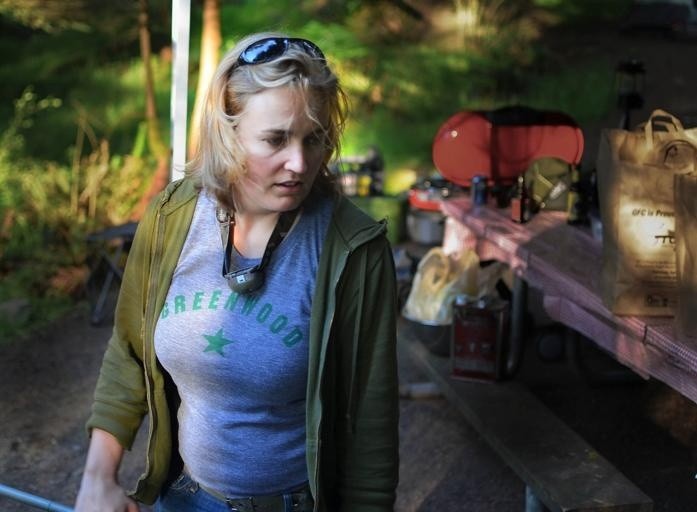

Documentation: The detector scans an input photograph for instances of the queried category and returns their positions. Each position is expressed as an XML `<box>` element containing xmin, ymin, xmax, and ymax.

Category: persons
<box><xmin>74</xmin><ymin>33</ymin><xmax>401</xmax><ymax>511</ymax></box>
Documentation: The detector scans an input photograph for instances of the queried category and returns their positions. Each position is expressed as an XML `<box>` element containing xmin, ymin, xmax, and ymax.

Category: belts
<box><xmin>225</xmin><ymin>481</ymin><xmax>311</xmax><ymax>511</ymax></box>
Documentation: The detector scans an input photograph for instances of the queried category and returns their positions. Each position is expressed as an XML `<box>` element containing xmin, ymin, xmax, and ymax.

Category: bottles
<box><xmin>511</xmin><ymin>168</ymin><xmax>530</xmax><ymax>224</ymax></box>
<box><xmin>422</xmin><ymin>175</ymin><xmax>444</xmax><ymax>189</ymax></box>
<box><xmin>338</xmin><ymin>146</ymin><xmax>383</xmax><ymax>197</ymax></box>
<box><xmin>439</xmin><ymin>182</ymin><xmax>459</xmax><ymax>198</ymax></box>
<box><xmin>400</xmin><ymin>379</ymin><xmax>441</xmax><ymax>400</ymax></box>
<box><xmin>470</xmin><ymin>173</ymin><xmax>487</xmax><ymax>206</ymax></box>
<box><xmin>564</xmin><ymin>162</ymin><xmax>585</xmax><ymax>226</ymax></box>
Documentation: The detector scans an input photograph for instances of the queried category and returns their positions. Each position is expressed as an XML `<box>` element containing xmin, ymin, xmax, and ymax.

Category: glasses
<box><xmin>221</xmin><ymin>37</ymin><xmax>332</xmax><ymax>83</ymax></box>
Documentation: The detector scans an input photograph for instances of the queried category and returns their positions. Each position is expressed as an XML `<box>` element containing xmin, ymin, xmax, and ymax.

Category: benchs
<box><xmin>396</xmin><ymin>305</ymin><xmax>655</xmax><ymax>511</ymax></box>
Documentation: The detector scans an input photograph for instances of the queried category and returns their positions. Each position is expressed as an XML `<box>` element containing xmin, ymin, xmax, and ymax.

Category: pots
<box><xmin>405</xmin><ymin>185</ymin><xmax>444</xmax><ymax>245</ymax></box>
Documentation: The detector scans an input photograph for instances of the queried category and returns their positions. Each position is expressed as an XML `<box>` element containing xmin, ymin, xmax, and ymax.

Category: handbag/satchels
<box><xmin>449</xmin><ymin>294</ymin><xmax>509</xmax><ymax>386</ymax></box>
<box><xmin>595</xmin><ymin>108</ymin><xmax>697</xmax><ymax>352</ymax></box>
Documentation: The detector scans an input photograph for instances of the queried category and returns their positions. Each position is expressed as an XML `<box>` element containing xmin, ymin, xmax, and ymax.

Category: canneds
<box><xmin>473</xmin><ymin>176</ymin><xmax>488</xmax><ymax>206</ymax></box>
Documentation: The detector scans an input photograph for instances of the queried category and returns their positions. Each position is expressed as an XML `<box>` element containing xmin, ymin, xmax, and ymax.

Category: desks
<box><xmin>440</xmin><ymin>194</ymin><xmax>696</xmax><ymax>401</ymax></box>
